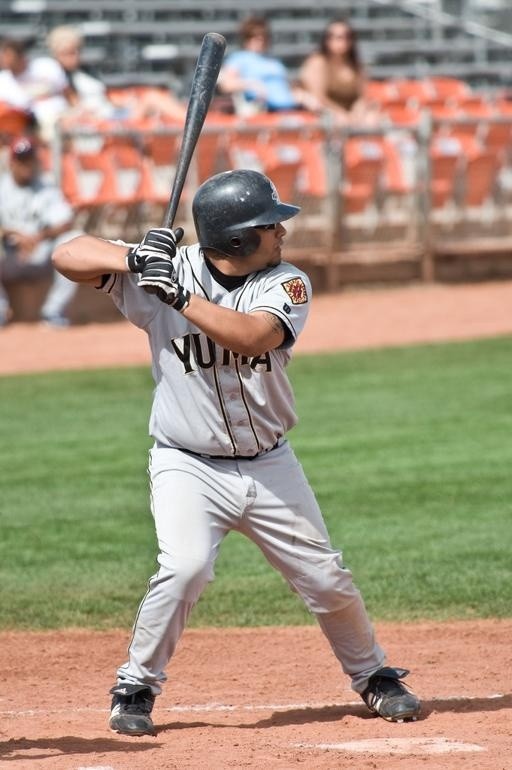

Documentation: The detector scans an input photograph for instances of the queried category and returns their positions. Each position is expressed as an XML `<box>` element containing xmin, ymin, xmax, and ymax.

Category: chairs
<box><xmin>0</xmin><ymin>75</ymin><xmax>512</xmax><ymax>321</ymax></box>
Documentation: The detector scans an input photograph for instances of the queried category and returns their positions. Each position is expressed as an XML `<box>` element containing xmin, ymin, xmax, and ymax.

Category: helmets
<box><xmin>192</xmin><ymin>169</ymin><xmax>301</xmax><ymax>257</ymax></box>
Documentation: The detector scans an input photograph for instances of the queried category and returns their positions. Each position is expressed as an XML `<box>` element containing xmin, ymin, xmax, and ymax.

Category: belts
<box><xmin>178</xmin><ymin>440</ymin><xmax>280</xmax><ymax>465</ymax></box>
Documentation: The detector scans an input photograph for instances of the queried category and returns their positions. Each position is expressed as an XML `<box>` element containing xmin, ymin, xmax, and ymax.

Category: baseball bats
<box><xmin>143</xmin><ymin>32</ymin><xmax>228</xmax><ymax>294</ymax></box>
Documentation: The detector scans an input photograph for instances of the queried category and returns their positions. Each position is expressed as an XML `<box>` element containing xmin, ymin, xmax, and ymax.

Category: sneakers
<box><xmin>360</xmin><ymin>666</ymin><xmax>421</xmax><ymax>722</ymax></box>
<box><xmin>109</xmin><ymin>685</ymin><xmax>156</xmax><ymax>735</ymax></box>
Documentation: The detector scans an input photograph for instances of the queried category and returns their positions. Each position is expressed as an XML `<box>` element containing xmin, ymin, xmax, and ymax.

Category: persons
<box><xmin>217</xmin><ymin>19</ymin><xmax>371</xmax><ymax>119</ymax></box>
<box><xmin>50</xmin><ymin>169</ymin><xmax>423</xmax><ymax>737</ymax></box>
<box><xmin>1</xmin><ymin>24</ymin><xmax>154</xmax><ymax>327</ymax></box>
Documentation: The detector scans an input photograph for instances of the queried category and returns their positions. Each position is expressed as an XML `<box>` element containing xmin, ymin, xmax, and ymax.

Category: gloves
<box><xmin>125</xmin><ymin>227</ymin><xmax>191</xmax><ymax>313</ymax></box>
